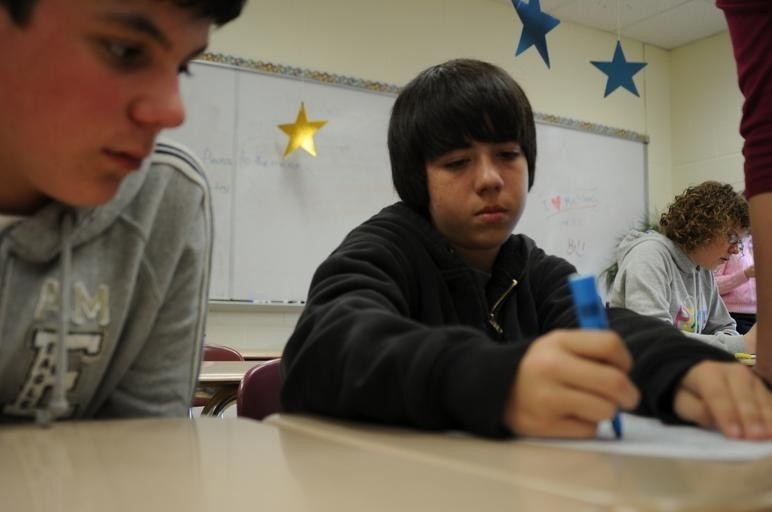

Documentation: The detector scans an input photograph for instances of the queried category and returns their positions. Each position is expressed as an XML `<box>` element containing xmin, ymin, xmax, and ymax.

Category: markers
<box><xmin>246</xmin><ymin>300</ymin><xmax>305</xmax><ymax>304</ymax></box>
<box><xmin>568</xmin><ymin>273</ymin><xmax>621</xmax><ymax>439</ymax></box>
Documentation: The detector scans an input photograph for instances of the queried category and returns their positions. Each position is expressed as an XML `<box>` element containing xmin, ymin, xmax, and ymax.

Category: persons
<box><xmin>0</xmin><ymin>0</ymin><xmax>250</xmax><ymax>424</ymax></box>
<box><xmin>604</xmin><ymin>177</ymin><xmax>757</xmax><ymax>361</ymax></box>
<box><xmin>710</xmin><ymin>189</ymin><xmax>758</xmax><ymax>335</ymax></box>
<box><xmin>714</xmin><ymin>0</ymin><xmax>771</xmax><ymax>390</ymax></box>
<box><xmin>277</xmin><ymin>57</ymin><xmax>771</xmax><ymax>443</ymax></box>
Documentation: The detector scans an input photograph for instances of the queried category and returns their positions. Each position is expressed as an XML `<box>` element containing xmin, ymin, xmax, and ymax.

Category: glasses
<box><xmin>724</xmin><ymin>230</ymin><xmax>745</xmax><ymax>252</ymax></box>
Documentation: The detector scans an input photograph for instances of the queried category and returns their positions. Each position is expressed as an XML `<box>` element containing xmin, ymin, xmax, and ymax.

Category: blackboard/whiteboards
<box><xmin>153</xmin><ymin>51</ymin><xmax>649</xmax><ymax>308</ymax></box>
